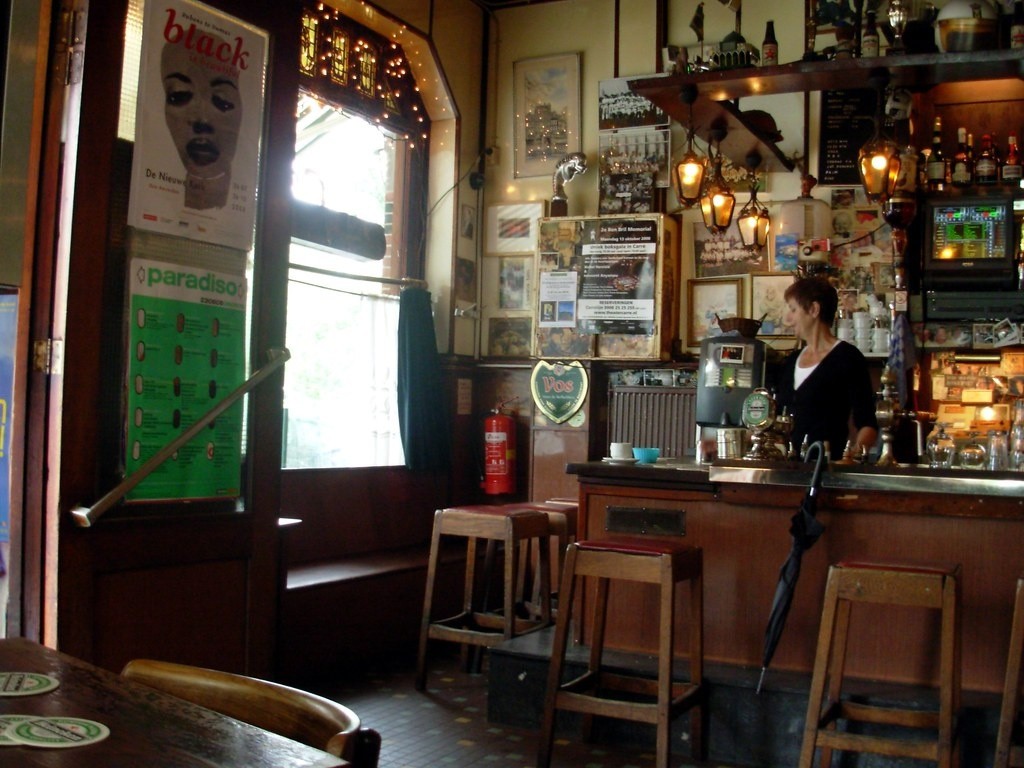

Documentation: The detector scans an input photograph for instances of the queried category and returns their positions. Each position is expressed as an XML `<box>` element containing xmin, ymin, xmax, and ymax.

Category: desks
<box><xmin>0</xmin><ymin>633</ymin><xmax>349</xmax><ymax>768</ymax></box>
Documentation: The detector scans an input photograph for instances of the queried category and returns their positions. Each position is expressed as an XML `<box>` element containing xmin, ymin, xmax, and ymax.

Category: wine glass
<box><xmin>926</xmin><ymin>421</ymin><xmax>956</xmax><ymax>469</ymax></box>
<box><xmin>959</xmin><ymin>431</ymin><xmax>989</xmax><ymax>471</ymax></box>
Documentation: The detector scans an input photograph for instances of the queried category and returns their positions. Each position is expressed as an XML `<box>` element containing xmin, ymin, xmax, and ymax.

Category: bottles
<box><xmin>919</xmin><ymin>117</ymin><xmax>1024</xmax><ymax>189</ymax></box>
<box><xmin>863</xmin><ymin>10</ymin><xmax>879</xmax><ymax>58</ymax></box>
<box><xmin>762</xmin><ymin>21</ymin><xmax>778</xmax><ymax>67</ymax></box>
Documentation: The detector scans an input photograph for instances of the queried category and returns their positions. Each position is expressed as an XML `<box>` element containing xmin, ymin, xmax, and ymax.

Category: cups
<box><xmin>987</xmin><ymin>398</ymin><xmax>1024</xmax><ymax>471</ymax></box>
<box><xmin>610</xmin><ymin>442</ymin><xmax>633</xmax><ymax>459</ymax></box>
<box><xmin>695</xmin><ymin>438</ymin><xmax>718</xmax><ymax>466</ymax></box>
<box><xmin>837</xmin><ymin>301</ymin><xmax>891</xmax><ymax>354</ymax></box>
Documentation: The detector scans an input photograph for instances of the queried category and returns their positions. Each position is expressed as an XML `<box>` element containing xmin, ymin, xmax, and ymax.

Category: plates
<box><xmin>603</xmin><ymin>457</ymin><xmax>640</xmax><ymax>464</ymax></box>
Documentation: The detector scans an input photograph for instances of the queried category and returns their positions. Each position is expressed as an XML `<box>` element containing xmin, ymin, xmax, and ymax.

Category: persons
<box><xmin>836</xmin><ymin>190</ymin><xmax>854</xmax><ymax>205</ymax></box>
<box><xmin>771</xmin><ymin>276</ymin><xmax>880</xmax><ymax>461</ymax></box>
<box><xmin>599</xmin><ymin>91</ymin><xmax>663</xmax><ymax>120</ymax></box>
<box><xmin>832</xmin><ymin>211</ymin><xmax>852</xmax><ymax>238</ymax></box>
<box><xmin>542</xmin><ymin>328</ymin><xmax>589</xmax><ymax>357</ymax></box>
<box><xmin>463</xmin><ymin>207</ymin><xmax>473</xmax><ymax>240</ymax></box>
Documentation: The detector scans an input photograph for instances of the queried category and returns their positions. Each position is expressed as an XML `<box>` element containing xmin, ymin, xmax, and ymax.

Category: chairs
<box><xmin>122</xmin><ymin>659</ymin><xmax>381</xmax><ymax>768</ymax></box>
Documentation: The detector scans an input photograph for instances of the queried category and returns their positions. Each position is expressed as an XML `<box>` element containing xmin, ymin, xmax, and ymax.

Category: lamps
<box><xmin>675</xmin><ymin>125</ymin><xmax>735</xmax><ymax>239</ymax></box>
<box><xmin>737</xmin><ymin>170</ymin><xmax>772</xmax><ymax>249</ymax></box>
<box><xmin>854</xmin><ymin>130</ymin><xmax>903</xmax><ymax>206</ymax></box>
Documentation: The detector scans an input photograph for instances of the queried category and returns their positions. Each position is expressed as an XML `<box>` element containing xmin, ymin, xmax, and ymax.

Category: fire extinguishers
<box><xmin>484</xmin><ymin>395</ymin><xmax>519</xmax><ymax>503</ymax></box>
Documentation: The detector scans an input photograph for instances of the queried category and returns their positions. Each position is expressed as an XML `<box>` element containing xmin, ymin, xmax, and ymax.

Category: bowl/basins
<box><xmin>631</xmin><ymin>447</ymin><xmax>659</xmax><ymax>463</ymax></box>
<box><xmin>939</xmin><ymin>19</ymin><xmax>998</xmax><ymax>52</ymax></box>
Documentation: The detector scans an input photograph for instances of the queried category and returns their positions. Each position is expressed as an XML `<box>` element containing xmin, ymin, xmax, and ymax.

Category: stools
<box><xmin>414</xmin><ymin>505</ymin><xmax>550</xmax><ymax>696</ymax></box>
<box><xmin>538</xmin><ymin>540</ymin><xmax>706</xmax><ymax>768</ymax></box>
<box><xmin>800</xmin><ymin>561</ymin><xmax>960</xmax><ymax>768</ymax></box>
<box><xmin>473</xmin><ymin>500</ymin><xmax>578</xmax><ymax>674</ymax></box>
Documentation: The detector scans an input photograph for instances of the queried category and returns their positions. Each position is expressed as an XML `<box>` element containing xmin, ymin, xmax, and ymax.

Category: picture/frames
<box><xmin>486</xmin><ymin>318</ymin><xmax>534</xmax><ymax>359</ymax></box>
<box><xmin>511</xmin><ymin>51</ymin><xmax>584</xmax><ymax>180</ymax></box>
<box><xmin>480</xmin><ymin>200</ymin><xmax>547</xmax><ymax>257</ymax></box>
